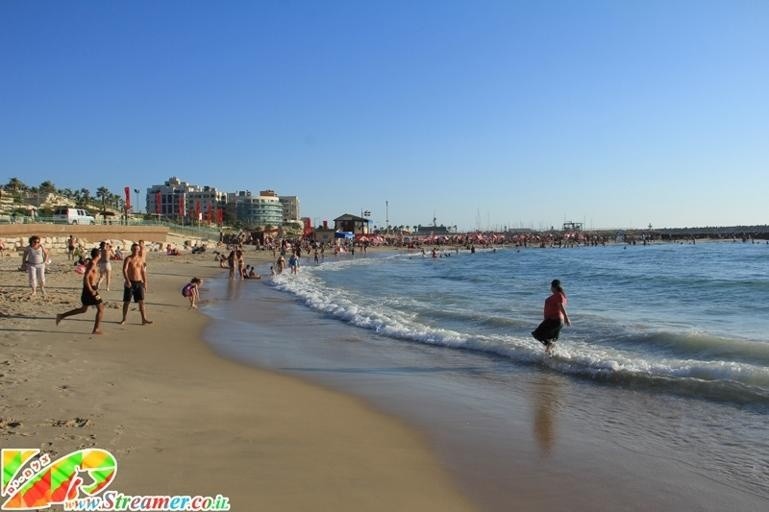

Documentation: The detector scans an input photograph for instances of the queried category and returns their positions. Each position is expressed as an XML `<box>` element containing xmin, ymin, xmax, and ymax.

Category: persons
<box><xmin>68</xmin><ymin>235</ymin><xmax>126</xmax><ymax>268</ymax></box>
<box><xmin>23</xmin><ymin>236</ymin><xmax>49</xmax><ymax>296</ymax></box>
<box><xmin>531</xmin><ymin>280</ymin><xmax>571</xmax><ymax>352</ymax></box>
<box><xmin>133</xmin><ymin>240</ymin><xmax>146</xmax><ymax>274</ymax></box>
<box><xmin>56</xmin><ymin>248</ymin><xmax>105</xmax><ymax>335</ymax></box>
<box><xmin>97</xmin><ymin>242</ymin><xmax>112</xmax><ymax>292</ymax></box>
<box><xmin>182</xmin><ymin>277</ymin><xmax>203</xmax><ymax>308</ymax></box>
<box><xmin>119</xmin><ymin>244</ymin><xmax>153</xmax><ymax>326</ymax></box>
<box><xmin>163</xmin><ymin>228</ymin><xmax>769</xmax><ymax>279</ymax></box>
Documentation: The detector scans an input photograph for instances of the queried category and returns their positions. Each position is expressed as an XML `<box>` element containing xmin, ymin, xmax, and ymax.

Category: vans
<box><xmin>52</xmin><ymin>206</ymin><xmax>95</xmax><ymax>226</ymax></box>
